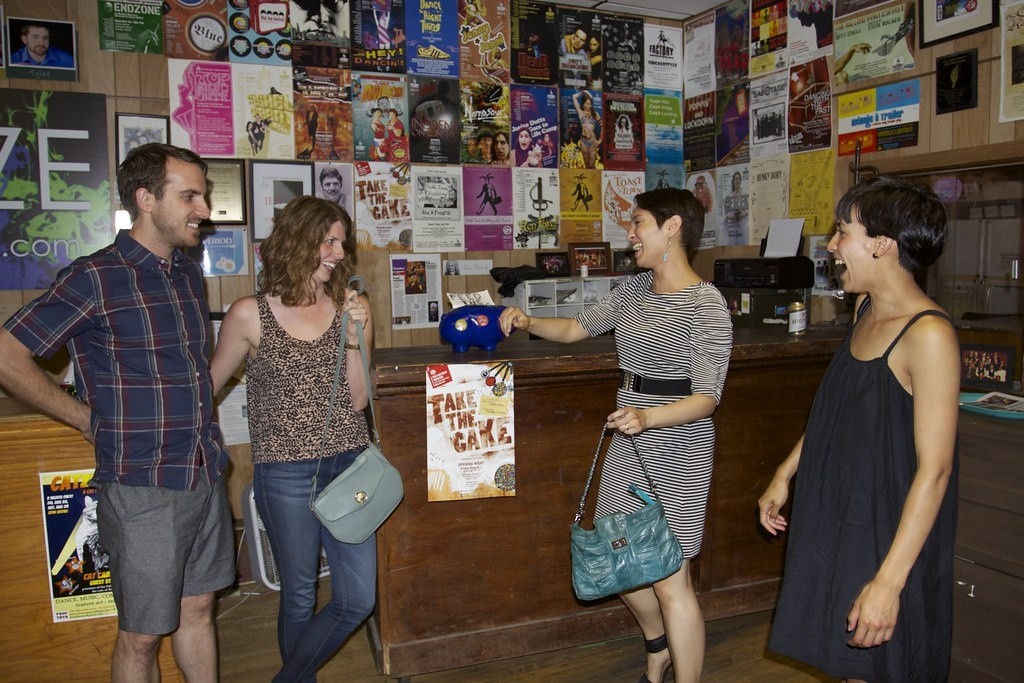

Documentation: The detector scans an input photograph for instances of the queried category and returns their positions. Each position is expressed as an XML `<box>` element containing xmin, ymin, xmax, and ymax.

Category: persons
<box><xmin>871</xmin><ymin>3</ymin><xmax>914</xmax><ymax>56</ymax></box>
<box><xmin>316</xmin><ymin>166</ymin><xmax>352</xmax><ymax>218</ymax></box>
<box><xmin>834</xmin><ymin>40</ymin><xmax>871</xmax><ymax>86</ymax></box>
<box><xmin>558</xmin><ymin>27</ymin><xmax>602</xmax><ymax>90</ymax></box>
<box><xmin>246</xmin><ymin>112</ymin><xmax>267</xmax><ymax>157</ymax></box>
<box><xmin>497</xmin><ymin>186</ymin><xmax>733</xmax><ymax>683</ymax></box>
<box><xmin>753</xmin><ymin>176</ymin><xmax>960</xmax><ymax>683</ymax></box>
<box><xmin>289</xmin><ymin>0</ymin><xmax>350</xmax><ymax>38</ymax></box>
<box><xmin>755</xmin><ymin>112</ymin><xmax>783</xmax><ymax>140</ymax></box>
<box><xmin>965</xmin><ymin>349</ymin><xmax>1006</xmax><ymax>383</ymax></box>
<box><xmin>723</xmin><ymin>171</ymin><xmax>749</xmax><ymax>246</ymax></box>
<box><xmin>10</xmin><ymin>22</ymin><xmax>75</xmax><ymax>69</ymax></box>
<box><xmin>689</xmin><ymin>175</ymin><xmax>713</xmax><ymax>213</ymax></box>
<box><xmin>571</xmin><ymin>88</ymin><xmax>604</xmax><ymax>170</ymax></box>
<box><xmin>213</xmin><ymin>194</ymin><xmax>379</xmax><ymax>683</ymax></box>
<box><xmin>512</xmin><ymin>126</ymin><xmax>550</xmax><ymax>168</ymax></box>
<box><xmin>0</xmin><ymin>141</ymin><xmax>237</xmax><ymax>683</ymax></box>
<box><xmin>462</xmin><ymin>128</ymin><xmax>512</xmax><ymax>167</ymax></box>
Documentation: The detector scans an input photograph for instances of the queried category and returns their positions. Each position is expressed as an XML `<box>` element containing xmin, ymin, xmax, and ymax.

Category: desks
<box><xmin>955</xmin><ymin>315</ymin><xmax>1024</xmax><ymax>380</ymax></box>
<box><xmin>1</xmin><ymin>396</ymin><xmax>188</xmax><ymax>683</ymax></box>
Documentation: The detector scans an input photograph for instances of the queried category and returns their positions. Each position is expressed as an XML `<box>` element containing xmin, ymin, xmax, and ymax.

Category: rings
<box><xmin>625</xmin><ymin>424</ymin><xmax>629</xmax><ymax>430</ymax></box>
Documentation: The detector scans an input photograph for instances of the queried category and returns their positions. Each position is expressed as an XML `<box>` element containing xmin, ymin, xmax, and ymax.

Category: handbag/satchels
<box><xmin>570</xmin><ymin>422</ymin><xmax>683</xmax><ymax>600</ymax></box>
<box><xmin>310</xmin><ymin>443</ymin><xmax>404</xmax><ymax>544</ymax></box>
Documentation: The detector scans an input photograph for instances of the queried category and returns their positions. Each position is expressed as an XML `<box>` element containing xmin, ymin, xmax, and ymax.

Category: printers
<box><xmin>712</xmin><ymin>224</ymin><xmax>815</xmax><ymax>286</ymax></box>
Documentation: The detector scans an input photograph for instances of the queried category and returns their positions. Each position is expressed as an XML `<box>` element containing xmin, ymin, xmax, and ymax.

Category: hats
<box><xmin>476</xmin><ymin>127</ymin><xmax>495</xmax><ymax>146</ymax></box>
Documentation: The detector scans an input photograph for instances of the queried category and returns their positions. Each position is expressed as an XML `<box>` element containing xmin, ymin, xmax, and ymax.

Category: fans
<box><xmin>241</xmin><ymin>482</ymin><xmax>330</xmax><ymax>591</ymax></box>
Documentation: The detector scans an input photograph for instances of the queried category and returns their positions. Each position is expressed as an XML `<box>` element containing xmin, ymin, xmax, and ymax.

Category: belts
<box><xmin>618</xmin><ymin>370</ymin><xmax>692</xmax><ymax>396</ymax></box>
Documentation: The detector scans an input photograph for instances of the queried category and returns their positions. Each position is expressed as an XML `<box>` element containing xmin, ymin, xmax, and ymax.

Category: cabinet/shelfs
<box><xmin>502</xmin><ymin>274</ymin><xmax>634</xmax><ymax>317</ymax></box>
<box><xmin>716</xmin><ymin>287</ymin><xmax>803</xmax><ymax>328</ymax></box>
<box><xmin>948</xmin><ymin>393</ymin><xmax>1024</xmax><ymax>683</ymax></box>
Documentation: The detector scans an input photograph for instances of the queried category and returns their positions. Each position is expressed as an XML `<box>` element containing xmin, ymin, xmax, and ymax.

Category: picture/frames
<box><xmin>613</xmin><ymin>251</ymin><xmax>652</xmax><ymax>274</ymax></box>
<box><xmin>115</xmin><ymin>111</ymin><xmax>170</xmax><ymax>176</ymax></box>
<box><xmin>248</xmin><ymin>158</ymin><xmax>315</xmax><ymax>243</ymax></box>
<box><xmin>958</xmin><ymin>343</ymin><xmax>1016</xmax><ymax>389</ymax></box>
<box><xmin>0</xmin><ymin>5</ymin><xmax>6</xmax><ymax>69</ymax></box>
<box><xmin>568</xmin><ymin>242</ymin><xmax>613</xmax><ymax>276</ymax></box>
<box><xmin>6</xmin><ymin>15</ymin><xmax>77</xmax><ymax>70</ymax></box>
<box><xmin>535</xmin><ymin>252</ymin><xmax>570</xmax><ymax>277</ymax></box>
<box><xmin>918</xmin><ymin>0</ymin><xmax>1001</xmax><ymax>49</ymax></box>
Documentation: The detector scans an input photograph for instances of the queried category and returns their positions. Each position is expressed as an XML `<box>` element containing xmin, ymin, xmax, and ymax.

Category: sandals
<box><xmin>636</xmin><ymin>634</ymin><xmax>671</xmax><ymax>683</ymax></box>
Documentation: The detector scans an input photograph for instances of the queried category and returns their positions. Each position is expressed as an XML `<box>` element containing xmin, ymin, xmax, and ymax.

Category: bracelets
<box><xmin>345</xmin><ymin>343</ymin><xmax>360</xmax><ymax>350</ymax></box>
